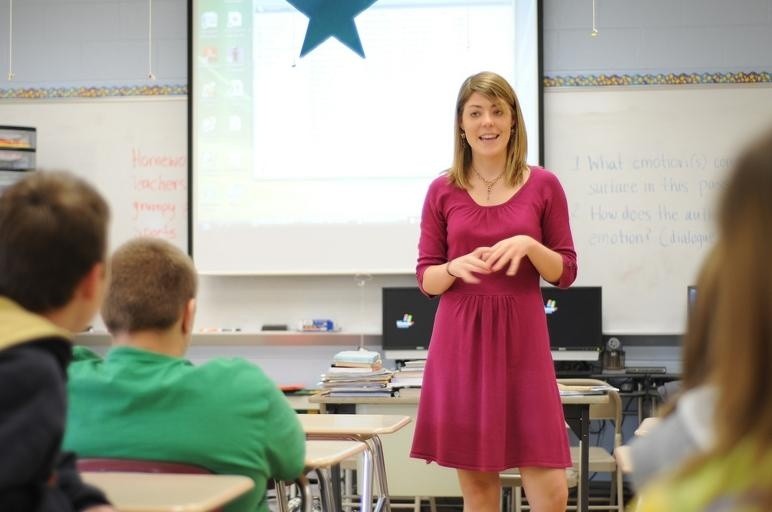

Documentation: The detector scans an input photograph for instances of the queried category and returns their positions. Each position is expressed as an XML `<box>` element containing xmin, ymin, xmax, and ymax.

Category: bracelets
<box><xmin>446</xmin><ymin>261</ymin><xmax>455</xmax><ymax>277</ymax></box>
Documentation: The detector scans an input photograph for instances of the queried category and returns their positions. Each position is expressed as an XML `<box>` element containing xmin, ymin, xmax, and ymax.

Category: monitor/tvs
<box><xmin>381</xmin><ymin>286</ymin><xmax>440</xmax><ymax>359</ymax></box>
<box><xmin>536</xmin><ymin>286</ymin><xmax>603</xmax><ymax>361</ymax></box>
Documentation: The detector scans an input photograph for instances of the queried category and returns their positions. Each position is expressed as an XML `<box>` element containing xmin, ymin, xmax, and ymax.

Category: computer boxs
<box><xmin>553</xmin><ymin>361</ymin><xmax>593</xmax><ymax>377</ymax></box>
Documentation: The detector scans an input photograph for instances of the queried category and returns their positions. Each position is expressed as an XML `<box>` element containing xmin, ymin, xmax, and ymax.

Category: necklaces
<box><xmin>470</xmin><ymin>165</ymin><xmax>507</xmax><ymax>201</ymax></box>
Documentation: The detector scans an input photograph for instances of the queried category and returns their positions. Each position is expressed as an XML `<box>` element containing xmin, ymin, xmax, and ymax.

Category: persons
<box><xmin>409</xmin><ymin>72</ymin><xmax>578</xmax><ymax>511</ymax></box>
<box><xmin>60</xmin><ymin>237</ymin><xmax>306</xmax><ymax>511</ymax></box>
<box><xmin>0</xmin><ymin>169</ymin><xmax>122</xmax><ymax>512</ymax></box>
<box><xmin>624</xmin><ymin>126</ymin><xmax>772</xmax><ymax>512</ymax></box>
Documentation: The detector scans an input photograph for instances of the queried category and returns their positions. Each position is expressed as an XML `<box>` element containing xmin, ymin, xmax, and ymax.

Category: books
<box><xmin>557</xmin><ymin>383</ymin><xmax>620</xmax><ymax>396</ymax></box>
<box><xmin>317</xmin><ymin>348</ymin><xmax>425</xmax><ymax>399</ymax></box>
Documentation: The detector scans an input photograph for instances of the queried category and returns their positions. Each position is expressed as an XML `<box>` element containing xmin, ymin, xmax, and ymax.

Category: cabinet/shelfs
<box><xmin>0</xmin><ymin>126</ymin><xmax>37</xmax><ymax>189</ymax></box>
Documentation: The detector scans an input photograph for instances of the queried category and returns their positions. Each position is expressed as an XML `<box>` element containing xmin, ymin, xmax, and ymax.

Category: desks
<box><xmin>79</xmin><ymin>371</ymin><xmax>684</xmax><ymax>512</ymax></box>
